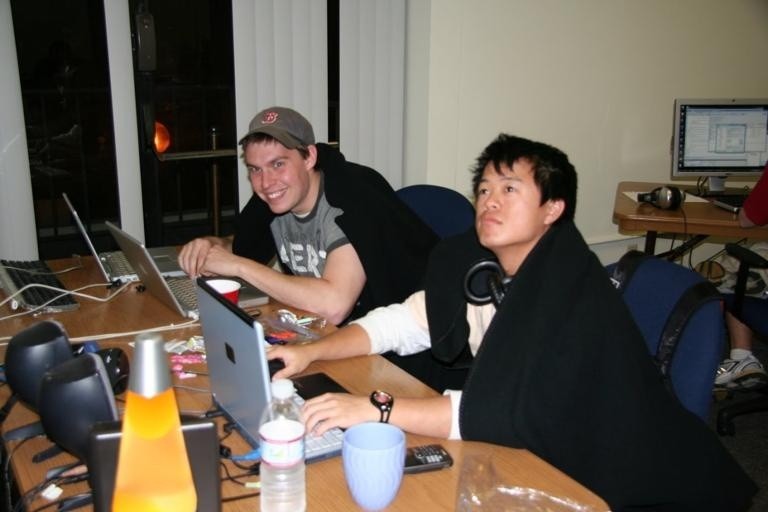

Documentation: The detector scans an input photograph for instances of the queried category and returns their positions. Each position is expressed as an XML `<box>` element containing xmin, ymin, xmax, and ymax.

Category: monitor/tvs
<box><xmin>671</xmin><ymin>98</ymin><xmax>768</xmax><ymax>198</ymax></box>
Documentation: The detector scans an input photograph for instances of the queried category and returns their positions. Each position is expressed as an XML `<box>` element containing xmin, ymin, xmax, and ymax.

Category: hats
<box><xmin>239</xmin><ymin>107</ymin><xmax>315</xmax><ymax>153</ymax></box>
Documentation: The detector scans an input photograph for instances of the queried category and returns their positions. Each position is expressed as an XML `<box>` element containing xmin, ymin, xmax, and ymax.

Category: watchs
<box><xmin>367</xmin><ymin>388</ymin><xmax>394</xmax><ymax>424</ymax></box>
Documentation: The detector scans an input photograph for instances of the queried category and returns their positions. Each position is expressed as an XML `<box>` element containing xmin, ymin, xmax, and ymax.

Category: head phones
<box><xmin>463</xmin><ymin>258</ymin><xmax>515</xmax><ymax>310</ymax></box>
<box><xmin>638</xmin><ymin>187</ymin><xmax>685</xmax><ymax>210</ymax></box>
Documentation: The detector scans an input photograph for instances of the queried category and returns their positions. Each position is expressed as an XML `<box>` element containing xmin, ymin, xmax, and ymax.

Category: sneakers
<box><xmin>711</xmin><ymin>353</ymin><xmax>766</xmax><ymax>392</ymax></box>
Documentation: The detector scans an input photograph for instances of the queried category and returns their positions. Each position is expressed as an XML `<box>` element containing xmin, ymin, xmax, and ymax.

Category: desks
<box><xmin>1</xmin><ymin>245</ymin><xmax>611</xmax><ymax>512</ymax></box>
<box><xmin>613</xmin><ymin>179</ymin><xmax>768</xmax><ymax>261</ymax></box>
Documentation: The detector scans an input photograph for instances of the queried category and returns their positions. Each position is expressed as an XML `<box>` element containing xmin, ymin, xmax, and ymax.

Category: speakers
<box><xmin>3</xmin><ymin>320</ymin><xmax>76</xmax><ymax>462</ymax></box>
<box><xmin>32</xmin><ymin>352</ymin><xmax>119</xmax><ymax>512</ymax></box>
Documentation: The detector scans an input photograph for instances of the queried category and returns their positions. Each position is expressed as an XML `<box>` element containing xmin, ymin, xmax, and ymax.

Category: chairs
<box><xmin>601</xmin><ymin>258</ymin><xmax>719</xmax><ymax>424</ymax></box>
<box><xmin>392</xmin><ymin>185</ymin><xmax>475</xmax><ymax>258</ymax></box>
<box><xmin>721</xmin><ymin>242</ymin><xmax>768</xmax><ymax>439</ymax></box>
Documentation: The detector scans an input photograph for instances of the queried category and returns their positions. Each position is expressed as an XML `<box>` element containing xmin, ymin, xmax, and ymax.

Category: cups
<box><xmin>203</xmin><ymin>279</ymin><xmax>242</xmax><ymax>309</ymax></box>
<box><xmin>341</xmin><ymin>423</ymin><xmax>407</xmax><ymax>510</ymax></box>
<box><xmin>92</xmin><ymin>347</ymin><xmax>130</xmax><ymax>394</ymax></box>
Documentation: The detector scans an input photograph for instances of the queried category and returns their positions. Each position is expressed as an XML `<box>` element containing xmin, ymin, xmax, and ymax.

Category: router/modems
<box><xmin>87</xmin><ymin>415</ymin><xmax>221</xmax><ymax>512</ymax></box>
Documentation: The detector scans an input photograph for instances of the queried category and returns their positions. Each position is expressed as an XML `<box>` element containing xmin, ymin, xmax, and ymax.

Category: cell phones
<box><xmin>403</xmin><ymin>444</ymin><xmax>452</xmax><ymax>474</ymax></box>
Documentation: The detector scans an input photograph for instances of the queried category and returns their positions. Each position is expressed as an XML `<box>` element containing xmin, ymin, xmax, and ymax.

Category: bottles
<box><xmin>255</xmin><ymin>376</ymin><xmax>308</xmax><ymax>512</ymax></box>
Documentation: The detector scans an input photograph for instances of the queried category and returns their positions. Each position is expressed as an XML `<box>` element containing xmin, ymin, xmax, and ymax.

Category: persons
<box><xmin>261</xmin><ymin>133</ymin><xmax>761</xmax><ymax>509</ymax></box>
<box><xmin>178</xmin><ymin>105</ymin><xmax>471</xmax><ymax>392</ymax></box>
<box><xmin>712</xmin><ymin>157</ymin><xmax>767</xmax><ymax>391</ymax></box>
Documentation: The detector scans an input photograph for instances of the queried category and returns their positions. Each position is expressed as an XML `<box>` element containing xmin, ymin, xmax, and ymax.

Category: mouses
<box><xmin>268</xmin><ymin>358</ymin><xmax>285</xmax><ymax>382</ymax></box>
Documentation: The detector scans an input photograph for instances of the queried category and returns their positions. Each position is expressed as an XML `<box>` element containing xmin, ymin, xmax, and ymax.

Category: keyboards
<box><xmin>713</xmin><ymin>193</ymin><xmax>749</xmax><ymax>214</ymax></box>
<box><xmin>0</xmin><ymin>260</ymin><xmax>80</xmax><ymax>313</ymax></box>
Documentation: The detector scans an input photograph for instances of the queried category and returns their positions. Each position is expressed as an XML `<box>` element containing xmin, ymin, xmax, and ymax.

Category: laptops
<box><xmin>105</xmin><ymin>221</ymin><xmax>269</xmax><ymax>319</ymax></box>
<box><xmin>62</xmin><ymin>192</ymin><xmax>188</xmax><ymax>283</ymax></box>
<box><xmin>192</xmin><ymin>277</ymin><xmax>357</xmax><ymax>472</ymax></box>
